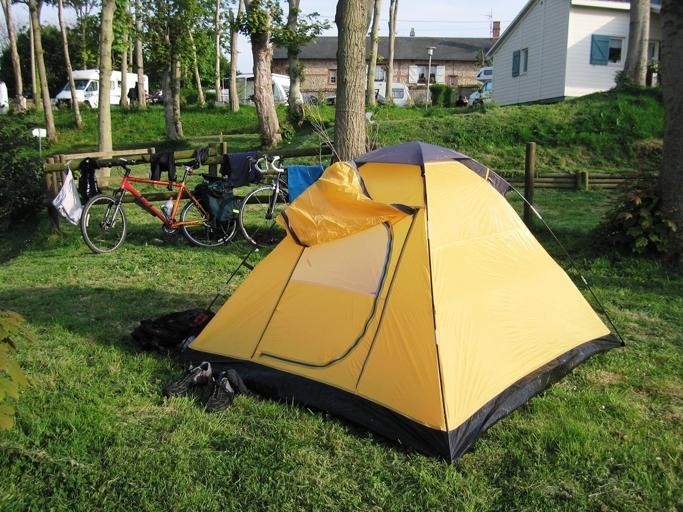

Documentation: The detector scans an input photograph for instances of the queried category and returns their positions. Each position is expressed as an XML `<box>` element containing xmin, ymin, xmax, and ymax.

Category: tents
<box><xmin>174</xmin><ymin>141</ymin><xmax>625</xmax><ymax>465</ymax></box>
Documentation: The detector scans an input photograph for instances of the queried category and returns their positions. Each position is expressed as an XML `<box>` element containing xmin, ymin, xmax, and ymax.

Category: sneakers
<box><xmin>204</xmin><ymin>372</ymin><xmax>235</xmax><ymax>413</ymax></box>
<box><xmin>164</xmin><ymin>361</ymin><xmax>213</xmax><ymax>399</ymax></box>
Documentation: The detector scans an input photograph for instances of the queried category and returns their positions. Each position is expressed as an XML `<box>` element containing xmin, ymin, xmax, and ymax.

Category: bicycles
<box><xmin>80</xmin><ymin>156</ymin><xmax>240</xmax><ymax>252</ymax></box>
<box><xmin>240</xmin><ymin>150</ymin><xmax>337</xmax><ymax>250</ymax></box>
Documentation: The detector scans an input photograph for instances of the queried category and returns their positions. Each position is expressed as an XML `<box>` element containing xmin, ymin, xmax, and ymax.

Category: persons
<box><xmin>456</xmin><ymin>96</ymin><xmax>464</xmax><ymax>107</ymax></box>
<box><xmin>463</xmin><ymin>96</ymin><xmax>468</xmax><ymax>102</ymax></box>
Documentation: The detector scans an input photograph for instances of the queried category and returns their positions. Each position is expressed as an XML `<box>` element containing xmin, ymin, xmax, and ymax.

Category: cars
<box><xmin>301</xmin><ymin>93</ymin><xmax>337</xmax><ymax>107</ymax></box>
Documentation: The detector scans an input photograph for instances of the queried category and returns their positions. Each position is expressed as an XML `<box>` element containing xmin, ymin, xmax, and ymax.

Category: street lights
<box><xmin>425</xmin><ymin>47</ymin><xmax>437</xmax><ymax>110</ymax></box>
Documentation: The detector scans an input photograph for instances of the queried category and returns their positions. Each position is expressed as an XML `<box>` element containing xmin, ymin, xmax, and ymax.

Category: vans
<box><xmin>367</xmin><ymin>82</ymin><xmax>414</xmax><ymax>107</ymax></box>
<box><xmin>468</xmin><ymin>67</ymin><xmax>492</xmax><ymax>108</ymax></box>
<box><xmin>56</xmin><ymin>69</ymin><xmax>150</xmax><ymax>113</ymax></box>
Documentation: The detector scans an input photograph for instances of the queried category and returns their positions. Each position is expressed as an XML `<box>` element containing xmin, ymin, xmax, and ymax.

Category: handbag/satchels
<box><xmin>52</xmin><ymin>170</ymin><xmax>84</xmax><ymax>227</ymax></box>
<box><xmin>194</xmin><ymin>182</ymin><xmax>236</xmax><ymax>221</ymax></box>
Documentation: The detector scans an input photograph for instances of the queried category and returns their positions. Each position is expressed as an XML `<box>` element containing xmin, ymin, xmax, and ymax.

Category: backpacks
<box><xmin>131</xmin><ymin>309</ymin><xmax>216</xmax><ymax>353</ymax></box>
